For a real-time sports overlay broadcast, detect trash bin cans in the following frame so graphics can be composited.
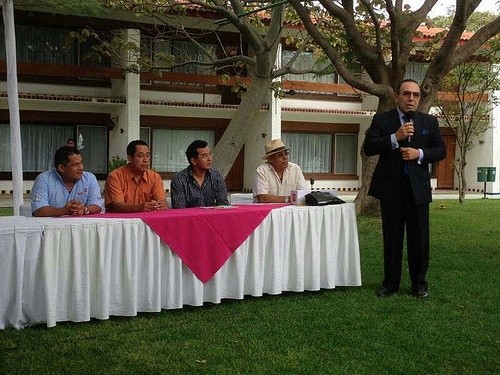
[477,166,496,183]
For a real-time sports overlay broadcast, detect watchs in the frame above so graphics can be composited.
[285,196,290,203]
[83,205,90,215]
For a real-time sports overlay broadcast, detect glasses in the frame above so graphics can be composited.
[197,151,214,158]
[397,91,421,98]
[274,151,289,158]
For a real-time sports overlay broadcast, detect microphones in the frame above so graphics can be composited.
[406,110,416,142]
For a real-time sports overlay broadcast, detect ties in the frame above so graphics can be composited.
[402,116,412,174]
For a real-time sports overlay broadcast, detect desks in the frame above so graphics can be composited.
[0,202,363,330]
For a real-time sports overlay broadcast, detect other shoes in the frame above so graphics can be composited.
[414,289,428,298]
[377,288,395,296]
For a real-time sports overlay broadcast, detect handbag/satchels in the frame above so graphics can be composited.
[305,191,334,205]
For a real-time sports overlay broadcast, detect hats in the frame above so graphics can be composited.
[262,138,290,159]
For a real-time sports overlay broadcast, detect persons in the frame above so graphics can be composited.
[363,79,447,298]
[67,138,76,147]
[30,145,105,217]
[171,140,229,209]
[252,139,305,204]
[103,140,169,214]
